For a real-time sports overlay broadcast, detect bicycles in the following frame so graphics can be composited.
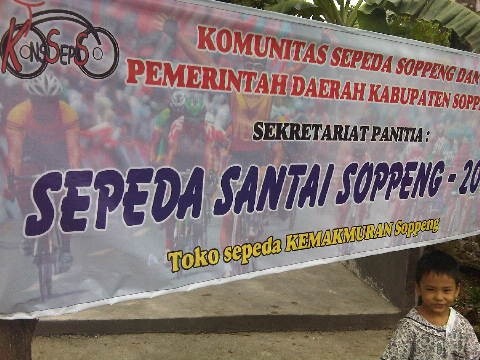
[223,166,480,280]
[166,168,215,266]
[8,165,69,301]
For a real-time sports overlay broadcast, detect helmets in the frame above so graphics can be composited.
[25,68,64,96]
[186,92,205,120]
[170,92,187,109]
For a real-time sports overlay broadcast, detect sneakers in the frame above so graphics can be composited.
[60,250,72,263]
[225,260,234,277]
[164,248,175,265]
[20,237,36,256]
[247,225,264,239]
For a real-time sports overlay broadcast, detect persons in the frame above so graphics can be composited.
[0,35,480,279]
[380,251,480,360]
